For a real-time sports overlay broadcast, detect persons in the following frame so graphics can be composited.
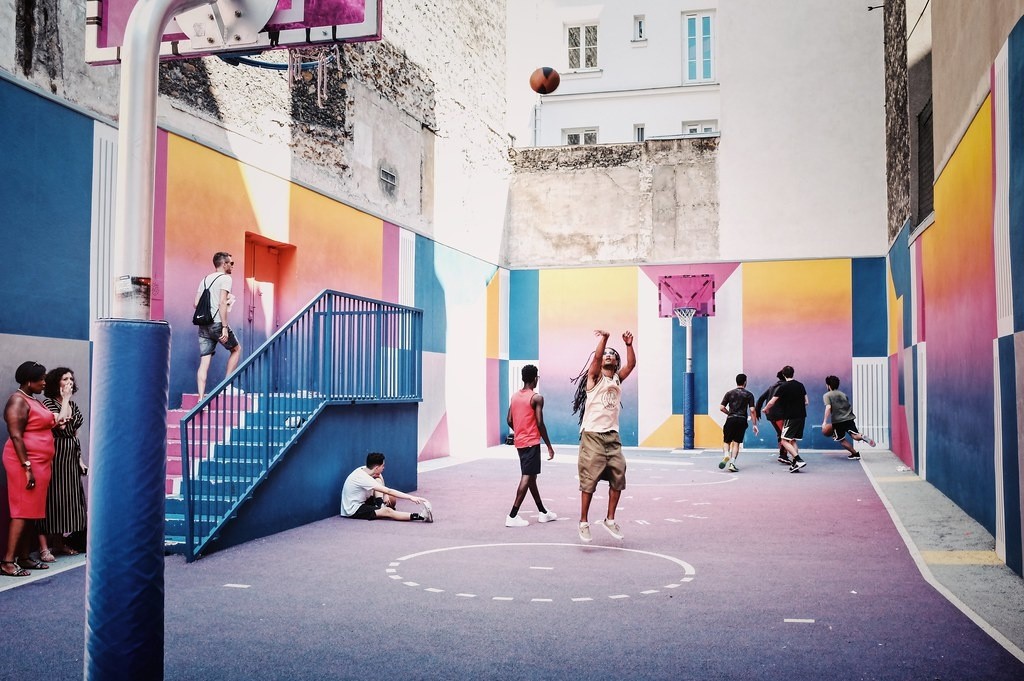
[720,374,759,471]
[504,365,557,527]
[341,453,434,523]
[0,361,56,577]
[570,330,636,543]
[822,375,876,459]
[42,367,89,561]
[194,252,245,410]
[755,370,792,464]
[762,366,809,472]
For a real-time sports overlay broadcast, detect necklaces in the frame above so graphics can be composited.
[17,388,36,400]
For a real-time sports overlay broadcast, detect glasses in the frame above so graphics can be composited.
[32,360,41,367]
[533,375,540,380]
[223,262,234,266]
[603,351,616,356]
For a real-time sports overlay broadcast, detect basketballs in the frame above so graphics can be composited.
[529,66,561,94]
[822,423,834,437]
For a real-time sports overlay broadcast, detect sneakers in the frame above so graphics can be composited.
[505,515,529,527]
[578,523,592,542]
[539,510,557,523]
[603,519,624,540]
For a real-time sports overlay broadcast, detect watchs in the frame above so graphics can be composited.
[22,461,31,467]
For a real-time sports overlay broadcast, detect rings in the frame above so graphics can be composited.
[32,483,34,486]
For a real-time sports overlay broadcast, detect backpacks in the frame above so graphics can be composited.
[192,274,226,325]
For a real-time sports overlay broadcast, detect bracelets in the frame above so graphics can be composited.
[626,343,632,346]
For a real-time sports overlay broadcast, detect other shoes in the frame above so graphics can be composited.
[718,456,730,469]
[60,545,78,555]
[782,465,799,473]
[39,549,56,562]
[848,452,860,460]
[197,401,208,411]
[220,385,244,396]
[420,502,434,522]
[728,463,739,472]
[861,435,876,447]
[790,460,806,473]
[778,455,792,465]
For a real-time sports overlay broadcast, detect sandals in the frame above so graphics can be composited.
[0,560,31,576]
[17,557,49,569]
